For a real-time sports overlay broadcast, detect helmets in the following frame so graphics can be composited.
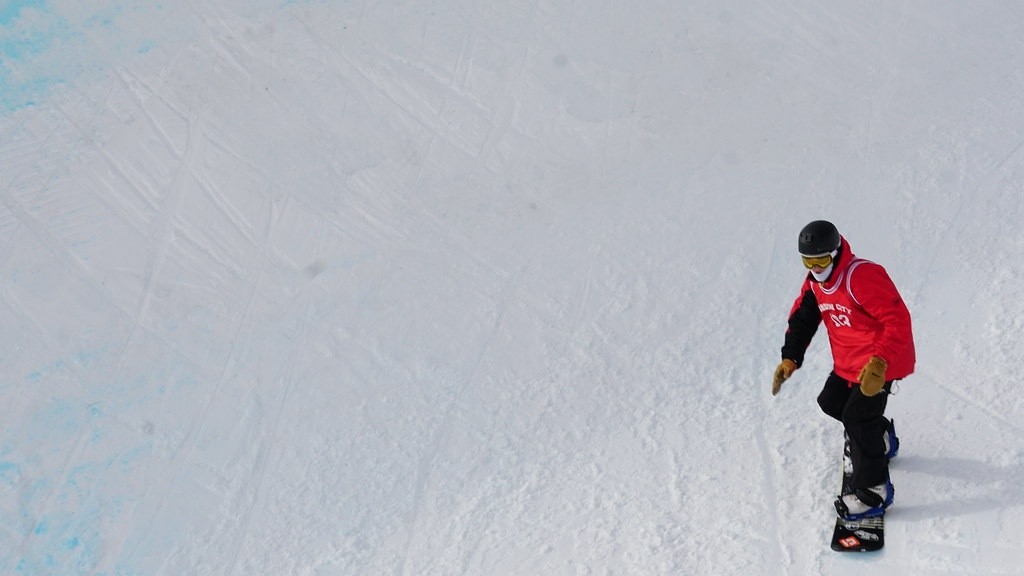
[797,220,840,256]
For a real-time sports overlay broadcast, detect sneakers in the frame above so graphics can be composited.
[842,437,892,465]
[840,478,894,516]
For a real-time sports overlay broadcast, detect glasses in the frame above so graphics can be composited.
[801,251,831,269]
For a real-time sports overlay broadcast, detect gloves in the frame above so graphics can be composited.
[857,356,887,396]
[771,358,797,395]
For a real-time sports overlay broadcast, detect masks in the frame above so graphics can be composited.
[810,264,833,283]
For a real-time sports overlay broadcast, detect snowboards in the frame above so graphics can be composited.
[828,420,888,553]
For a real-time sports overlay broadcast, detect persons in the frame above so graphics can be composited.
[772,220,917,518]
